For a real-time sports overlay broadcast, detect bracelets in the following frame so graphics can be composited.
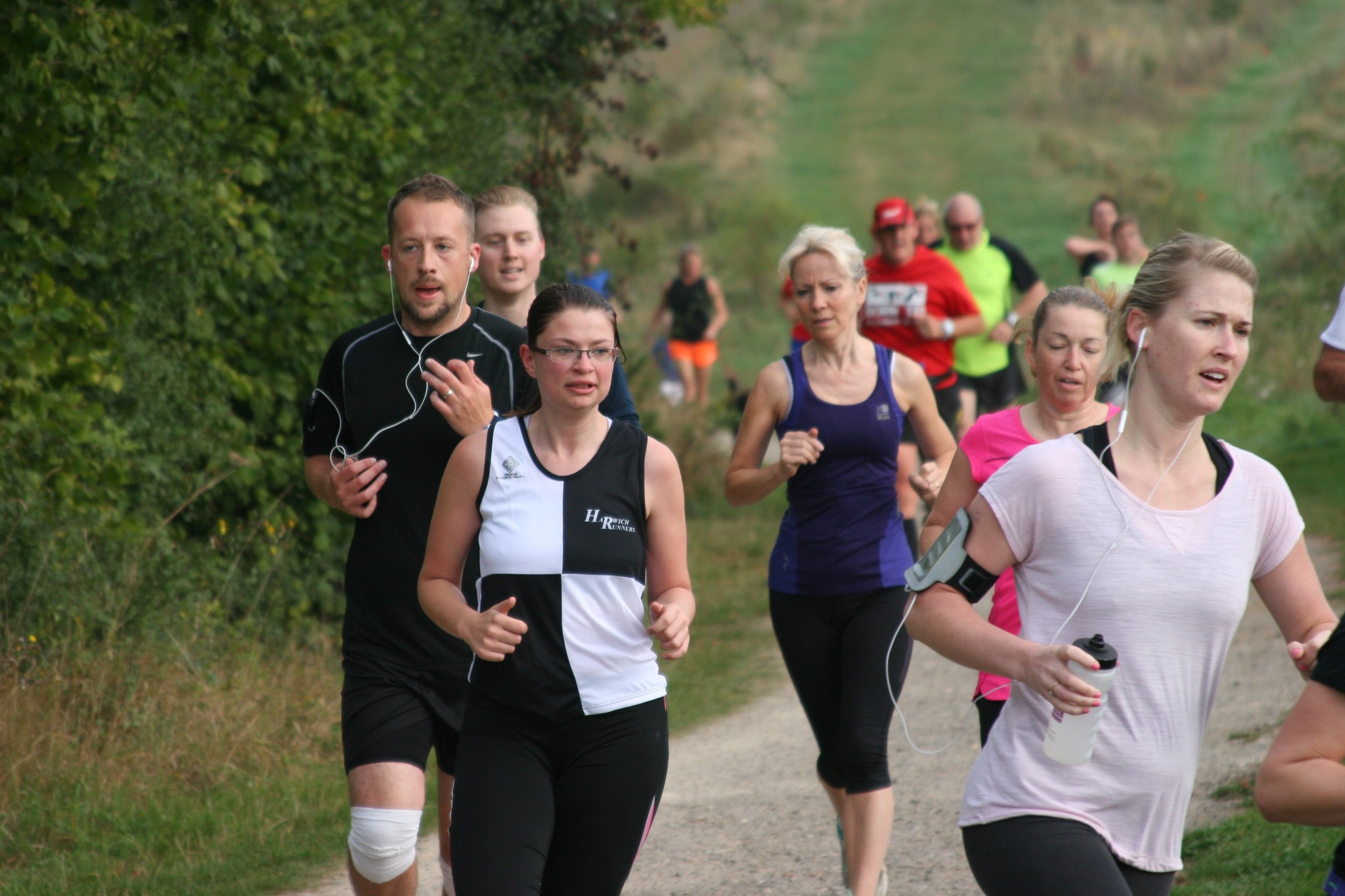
[940,317,955,341]
[484,409,499,430]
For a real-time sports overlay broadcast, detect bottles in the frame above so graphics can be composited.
[1041,633,1118,766]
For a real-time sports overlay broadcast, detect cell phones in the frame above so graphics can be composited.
[909,512,964,582]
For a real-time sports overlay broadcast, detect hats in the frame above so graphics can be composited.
[872,197,916,232]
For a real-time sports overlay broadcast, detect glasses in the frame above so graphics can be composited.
[945,221,983,232]
[530,346,621,364]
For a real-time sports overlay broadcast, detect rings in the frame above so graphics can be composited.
[442,389,453,399]
[1049,680,1061,697]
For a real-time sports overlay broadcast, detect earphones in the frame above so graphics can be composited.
[468,256,474,273]
[1137,324,1148,349]
[388,259,392,273]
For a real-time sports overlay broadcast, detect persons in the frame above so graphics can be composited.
[562,240,623,323]
[853,193,985,567]
[916,277,1131,752]
[469,184,642,429]
[1313,282,1345,403]
[724,222,959,895]
[415,279,696,896]
[1252,609,1345,896]
[644,245,731,415]
[776,186,1158,448]
[301,176,544,896]
[899,231,1341,896]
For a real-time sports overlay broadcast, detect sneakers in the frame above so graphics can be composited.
[835,814,889,896]
